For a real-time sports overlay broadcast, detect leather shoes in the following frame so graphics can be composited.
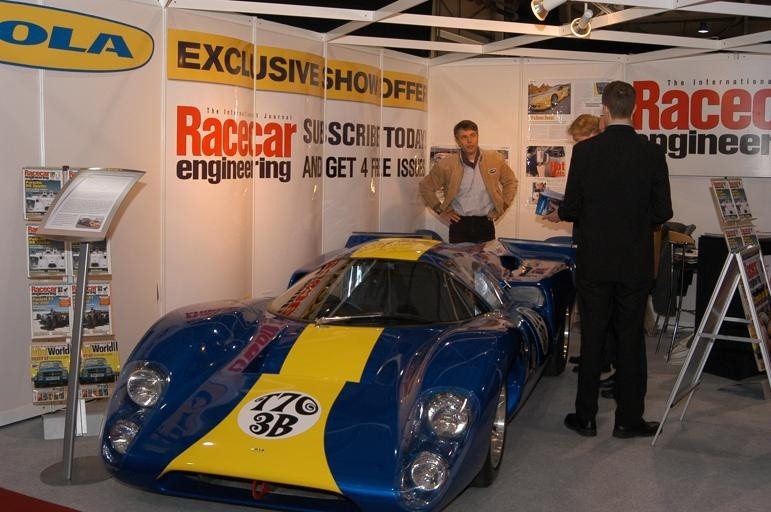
[613,422,662,438]
[564,414,597,436]
[569,356,615,399]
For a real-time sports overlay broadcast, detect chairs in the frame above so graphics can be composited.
[655,222,698,362]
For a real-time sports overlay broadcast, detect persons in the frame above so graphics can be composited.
[543,80,664,439]
[559,114,611,374]
[530,147,545,176]
[419,119,518,243]
[589,129,663,399]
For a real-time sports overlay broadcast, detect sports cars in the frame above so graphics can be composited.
[100,230,577,509]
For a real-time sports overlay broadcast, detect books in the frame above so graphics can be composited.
[535,189,566,215]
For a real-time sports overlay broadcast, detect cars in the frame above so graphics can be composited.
[34,360,68,387]
[79,358,115,385]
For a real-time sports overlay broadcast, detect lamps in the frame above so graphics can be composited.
[697,23,710,34]
[531,0,594,38]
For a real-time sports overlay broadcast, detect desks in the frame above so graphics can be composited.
[695,233,771,382]
